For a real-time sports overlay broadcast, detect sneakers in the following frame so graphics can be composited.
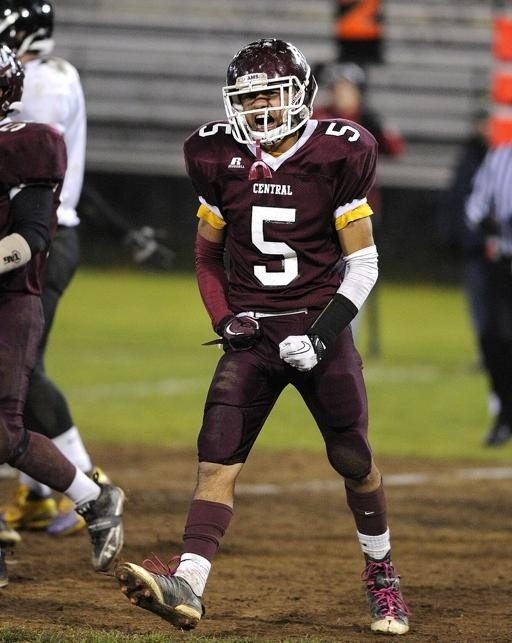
[360,550,412,635]
[1,464,204,631]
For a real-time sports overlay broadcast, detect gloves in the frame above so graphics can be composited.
[222,316,260,352]
[279,335,327,372]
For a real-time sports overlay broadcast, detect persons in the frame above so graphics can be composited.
[436,109,501,413]
[312,65,405,358]
[114,36,410,635]
[2,1,112,538]
[0,44,126,588]
[464,135,512,448]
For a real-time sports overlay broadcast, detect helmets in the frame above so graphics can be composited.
[221,37,319,145]
[0,41,24,116]
[0,0,54,55]
